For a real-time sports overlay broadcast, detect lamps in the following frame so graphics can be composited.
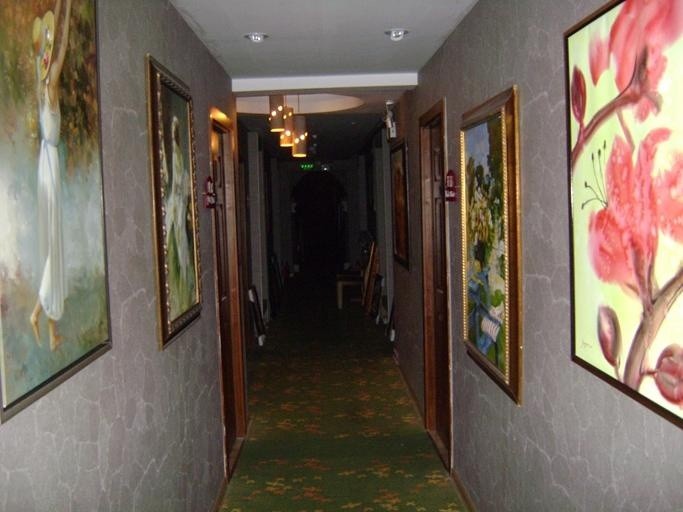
[265,87,309,160]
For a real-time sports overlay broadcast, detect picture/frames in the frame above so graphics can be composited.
[391,136,411,271]
[558,1,683,435]
[455,81,525,406]
[143,52,205,355]
[0,0,114,432]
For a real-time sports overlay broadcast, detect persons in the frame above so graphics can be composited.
[27,0,74,352]
[169,116,192,310]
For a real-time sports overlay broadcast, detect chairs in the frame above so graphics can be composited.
[334,241,374,309]
